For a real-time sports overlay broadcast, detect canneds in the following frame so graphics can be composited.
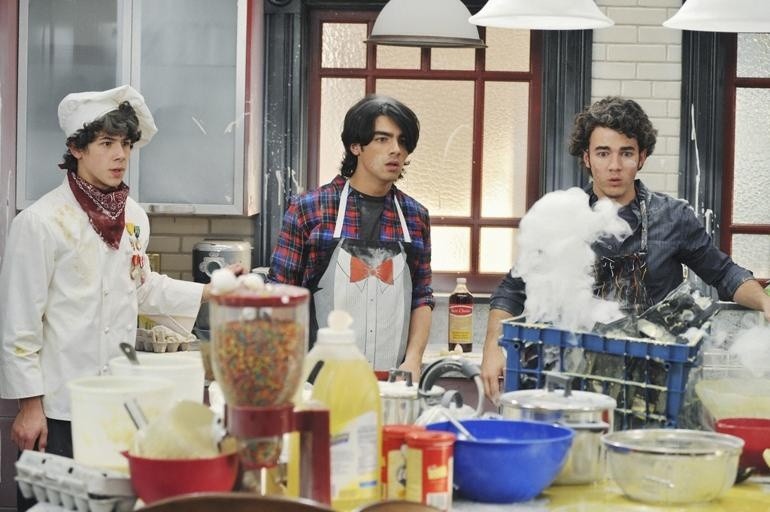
[405,429,454,510]
[382,424,423,501]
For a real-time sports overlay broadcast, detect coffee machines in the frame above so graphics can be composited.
[207,280,331,508]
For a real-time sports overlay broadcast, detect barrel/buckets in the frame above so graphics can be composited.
[293,328,383,510]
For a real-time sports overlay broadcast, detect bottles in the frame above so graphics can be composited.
[383,427,453,509]
[448,276,474,351]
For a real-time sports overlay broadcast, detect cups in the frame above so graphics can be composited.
[134,327,200,353]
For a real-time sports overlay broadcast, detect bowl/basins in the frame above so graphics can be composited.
[718,416,770,470]
[604,427,746,507]
[116,442,241,504]
[424,420,574,502]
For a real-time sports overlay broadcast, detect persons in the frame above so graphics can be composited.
[266,99,435,426]
[481,96,770,431]
[1,82,263,510]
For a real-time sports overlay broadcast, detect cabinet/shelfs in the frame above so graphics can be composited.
[15,2,264,218]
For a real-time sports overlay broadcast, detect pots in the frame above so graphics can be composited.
[496,372,619,482]
[375,369,446,425]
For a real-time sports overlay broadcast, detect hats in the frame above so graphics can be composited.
[57,86,158,149]
